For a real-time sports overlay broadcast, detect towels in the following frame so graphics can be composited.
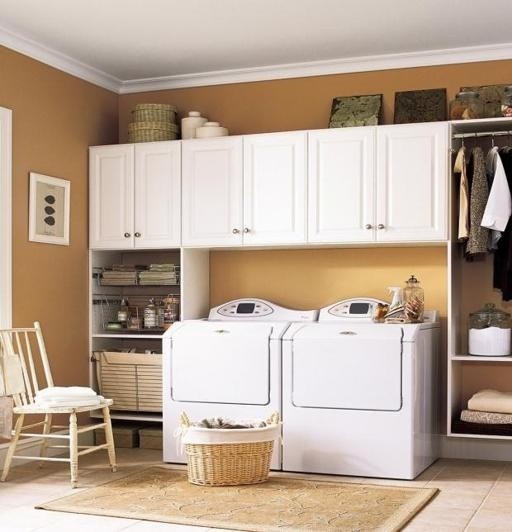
[34,385,103,410]
[0,330,26,396]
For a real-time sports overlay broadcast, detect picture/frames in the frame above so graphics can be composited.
[327,92,383,128]
[393,87,446,124]
[27,170,71,245]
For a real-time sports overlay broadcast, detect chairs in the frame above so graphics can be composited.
[0,321,118,488]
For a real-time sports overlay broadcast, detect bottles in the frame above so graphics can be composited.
[402,272,425,324]
[117,297,164,329]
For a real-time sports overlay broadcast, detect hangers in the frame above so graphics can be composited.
[461,129,510,148]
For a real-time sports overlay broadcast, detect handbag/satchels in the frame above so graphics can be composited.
[0,353,25,397]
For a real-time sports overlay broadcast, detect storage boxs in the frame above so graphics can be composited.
[138,428,163,450]
[94,426,140,449]
[94,351,162,413]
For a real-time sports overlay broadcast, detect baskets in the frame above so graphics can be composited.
[131,103,178,123]
[180,409,279,487]
[126,122,179,143]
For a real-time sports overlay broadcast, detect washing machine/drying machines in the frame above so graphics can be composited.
[162,297,318,470]
[281,298,442,480]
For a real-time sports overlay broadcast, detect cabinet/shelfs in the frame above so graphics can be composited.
[182,129,308,249]
[446,117,512,440]
[87,249,209,421]
[307,120,449,248]
[88,139,182,249]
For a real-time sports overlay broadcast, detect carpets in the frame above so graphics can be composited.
[33,465,439,532]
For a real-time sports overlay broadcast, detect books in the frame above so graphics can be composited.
[100,262,180,285]
